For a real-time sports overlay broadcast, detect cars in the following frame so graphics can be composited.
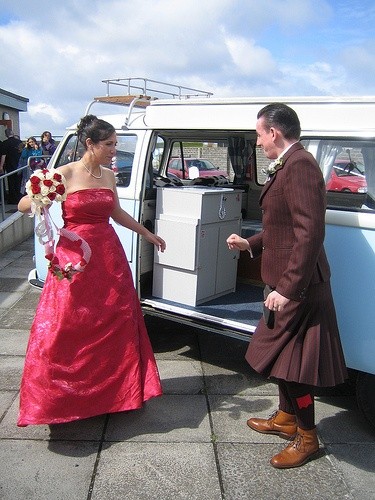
[326,158,368,197]
[164,155,232,185]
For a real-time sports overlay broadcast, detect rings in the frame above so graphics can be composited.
[273,306,278,309]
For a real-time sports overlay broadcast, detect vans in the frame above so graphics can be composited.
[27,73,374,415]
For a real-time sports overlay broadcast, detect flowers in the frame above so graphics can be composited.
[25,166,92,283]
[261,160,283,185]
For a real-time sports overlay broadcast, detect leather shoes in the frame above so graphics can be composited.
[270,426,319,468]
[247,410,297,440]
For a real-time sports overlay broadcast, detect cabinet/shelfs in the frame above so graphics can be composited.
[151,187,245,308]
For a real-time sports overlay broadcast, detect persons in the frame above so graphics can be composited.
[226,103,350,469]
[0,128,82,205]
[16,114,166,428]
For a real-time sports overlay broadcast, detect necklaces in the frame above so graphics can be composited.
[81,158,103,179]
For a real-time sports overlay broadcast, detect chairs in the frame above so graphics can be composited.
[152,177,184,188]
[189,175,229,186]
